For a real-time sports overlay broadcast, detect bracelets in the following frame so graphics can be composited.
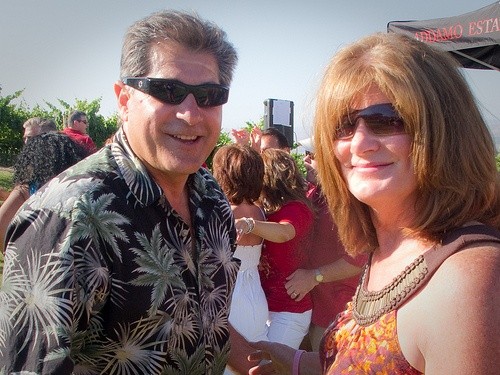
[258,360,269,366]
[248,217,256,232]
[242,216,252,234]
[292,350,307,375]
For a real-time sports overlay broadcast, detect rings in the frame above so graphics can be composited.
[293,292,298,296]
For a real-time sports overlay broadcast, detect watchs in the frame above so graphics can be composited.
[315,268,324,283]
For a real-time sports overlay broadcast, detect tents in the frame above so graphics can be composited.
[386,0,500,71]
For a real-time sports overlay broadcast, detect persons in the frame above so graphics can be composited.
[200,121,370,375]
[246,33,500,375]
[164,85,176,101]
[0,8,271,375]
[0,109,115,254]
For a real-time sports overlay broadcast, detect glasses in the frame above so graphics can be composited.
[78,120,87,124]
[124,77,229,107]
[334,103,410,140]
[305,150,318,159]
